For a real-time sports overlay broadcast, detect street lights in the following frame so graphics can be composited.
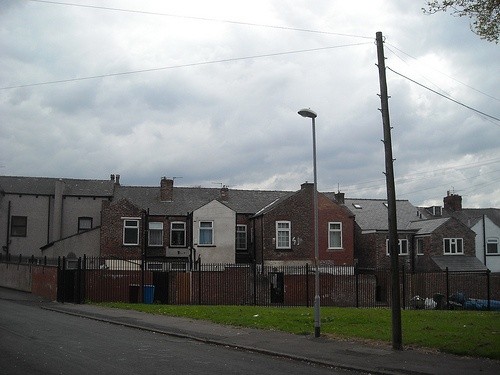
[297,109,321,340]
